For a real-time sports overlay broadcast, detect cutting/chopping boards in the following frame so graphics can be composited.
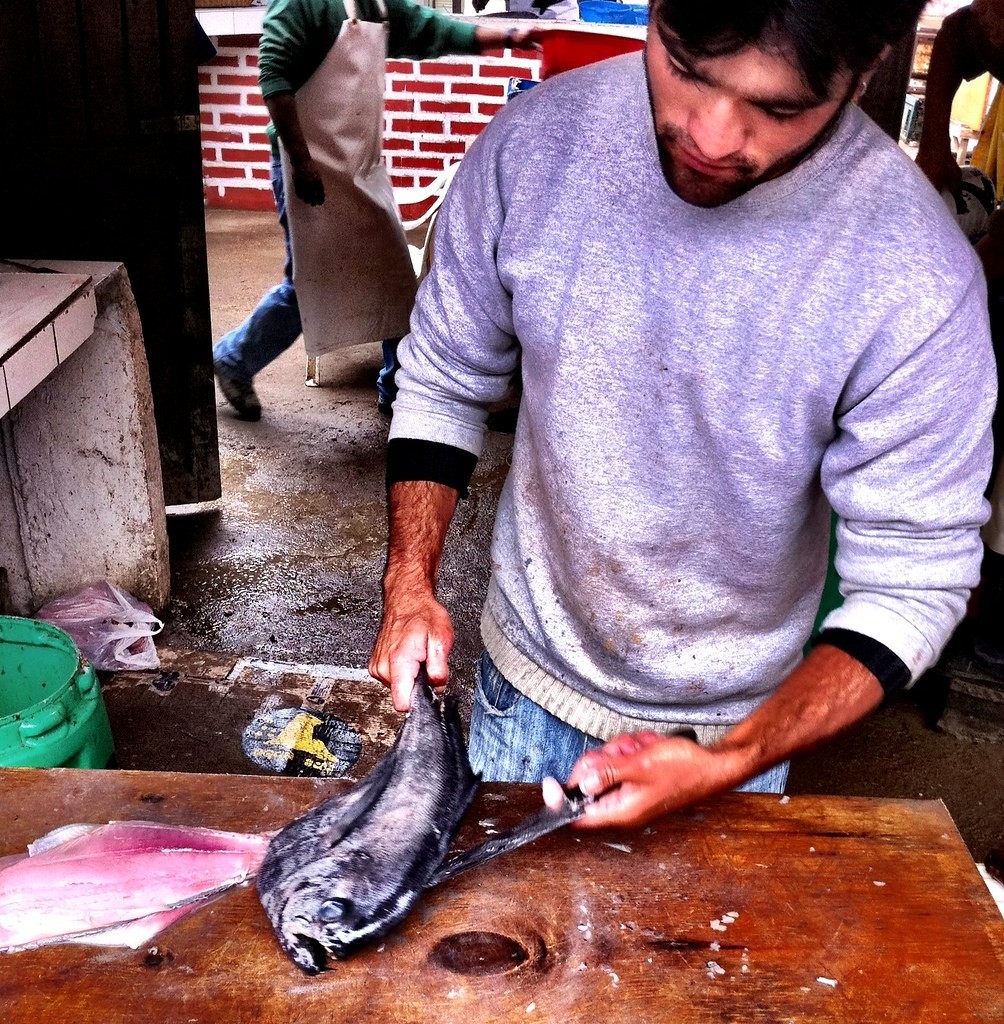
[0,767,1004,1024]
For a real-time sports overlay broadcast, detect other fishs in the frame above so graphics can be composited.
[256,662,483,977]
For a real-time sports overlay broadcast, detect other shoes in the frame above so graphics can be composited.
[213,354,261,416]
[378,401,393,412]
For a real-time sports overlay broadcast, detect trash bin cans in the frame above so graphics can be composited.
[0,613,115,770]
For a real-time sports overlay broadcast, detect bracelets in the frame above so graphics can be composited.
[505,28,517,49]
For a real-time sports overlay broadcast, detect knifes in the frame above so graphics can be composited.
[429,727,696,915]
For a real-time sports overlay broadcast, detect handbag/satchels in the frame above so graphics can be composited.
[38,581,166,670]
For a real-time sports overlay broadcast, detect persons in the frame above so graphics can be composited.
[212,0,558,420]
[367,0,1004,827]
